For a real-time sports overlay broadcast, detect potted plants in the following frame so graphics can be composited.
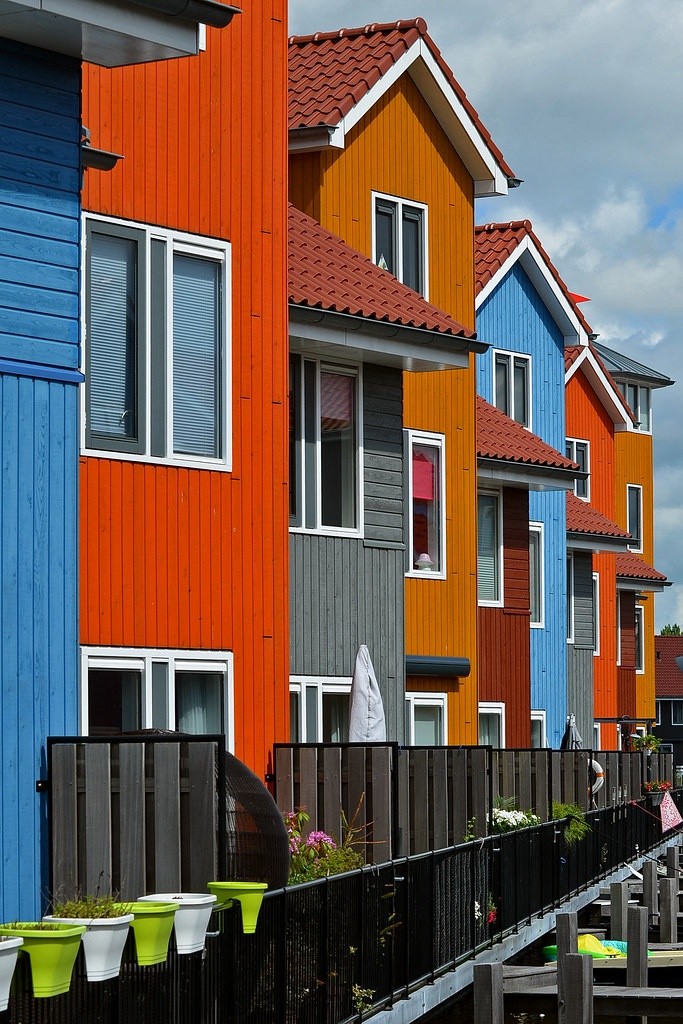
[0,917,86,998]
[42,870,134,982]
[649,791,666,807]
[633,734,664,755]
[0,934,24,1011]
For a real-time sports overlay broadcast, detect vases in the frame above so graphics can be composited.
[137,893,217,954]
[96,902,180,965]
[207,882,268,933]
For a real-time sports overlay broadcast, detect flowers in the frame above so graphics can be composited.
[640,780,671,791]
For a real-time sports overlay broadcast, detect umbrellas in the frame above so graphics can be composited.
[560,715,583,750]
[343,644,386,742]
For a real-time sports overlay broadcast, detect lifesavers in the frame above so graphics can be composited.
[588,758,606,796]
